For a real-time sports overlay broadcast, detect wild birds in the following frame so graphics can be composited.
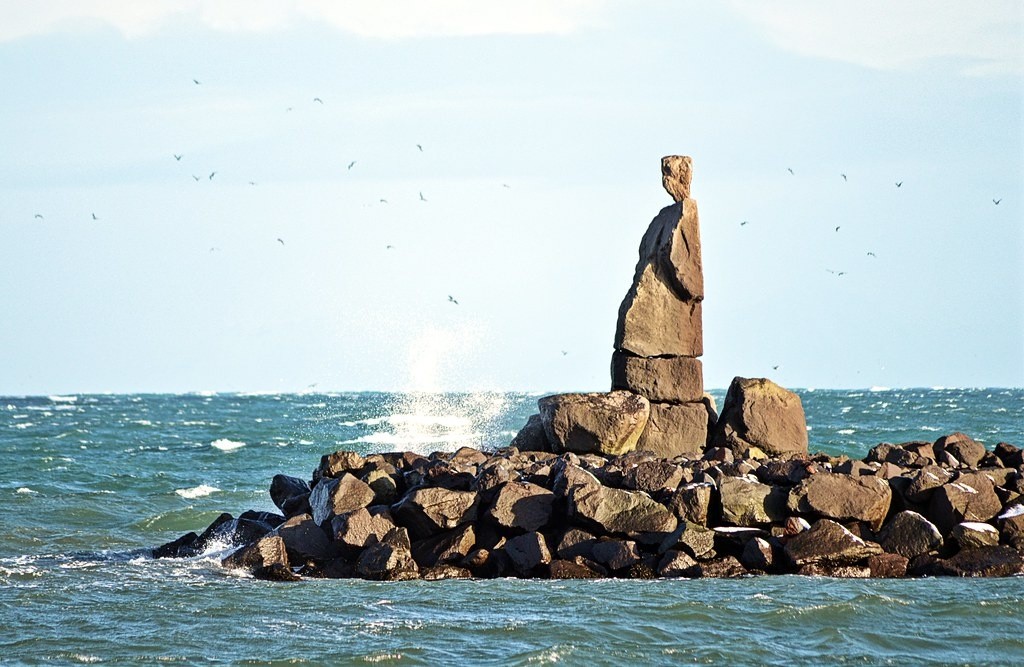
[740,164,1001,369]
[35,68,473,306]
